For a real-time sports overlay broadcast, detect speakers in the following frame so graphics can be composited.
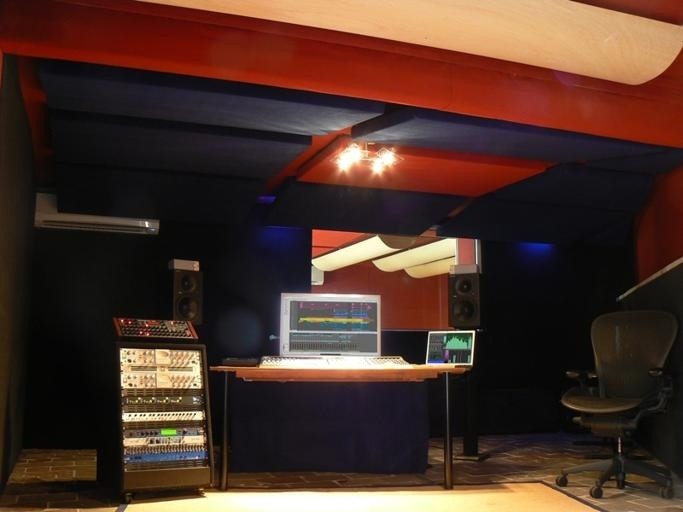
[447,272,481,328]
[172,269,204,326]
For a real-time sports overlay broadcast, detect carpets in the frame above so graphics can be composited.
[113,477,610,512]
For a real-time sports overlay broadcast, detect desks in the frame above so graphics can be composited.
[209,367,470,490]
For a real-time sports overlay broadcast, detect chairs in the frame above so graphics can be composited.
[555,308,676,501]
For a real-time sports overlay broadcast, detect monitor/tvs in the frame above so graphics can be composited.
[425,329,476,366]
[279,290,381,357]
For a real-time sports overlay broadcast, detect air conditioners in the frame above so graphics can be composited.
[33,192,160,237]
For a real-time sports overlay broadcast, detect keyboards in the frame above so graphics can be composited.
[258,353,414,370]
